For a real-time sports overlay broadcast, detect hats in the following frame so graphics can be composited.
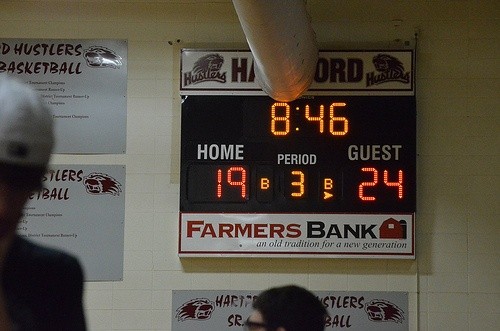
[0,75,53,166]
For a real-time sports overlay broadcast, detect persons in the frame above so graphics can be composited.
[246,285,327,331]
[0,74,88,331]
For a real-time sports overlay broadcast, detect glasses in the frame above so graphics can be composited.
[245,316,268,331]
